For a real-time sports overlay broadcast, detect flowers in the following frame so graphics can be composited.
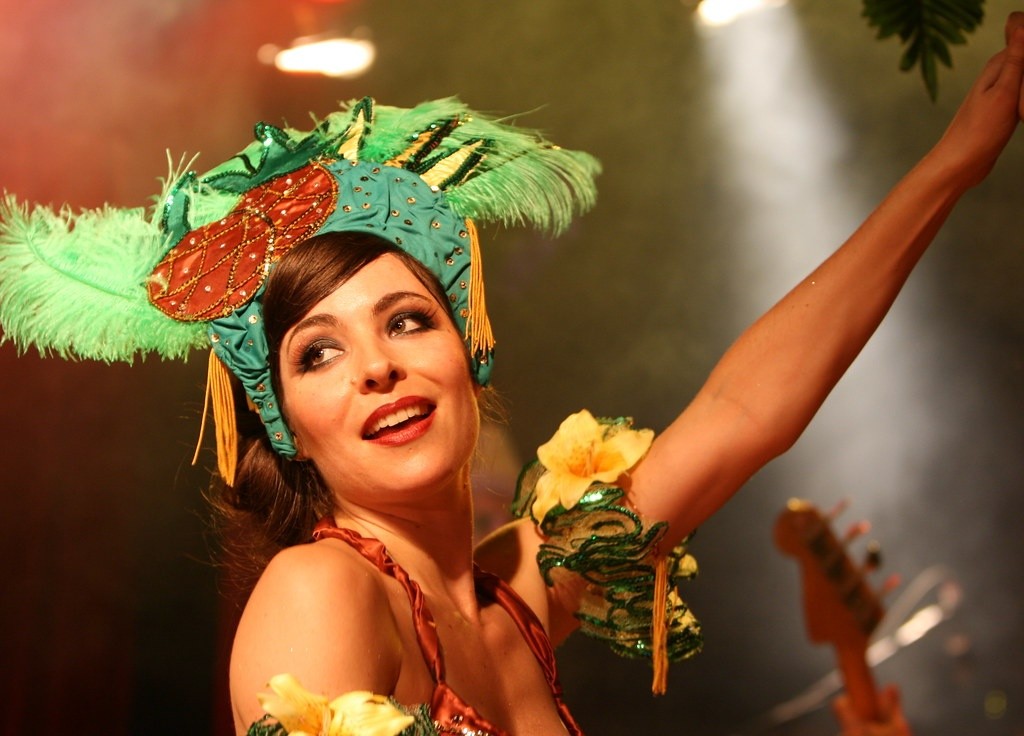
[253,674,417,736]
[526,407,655,527]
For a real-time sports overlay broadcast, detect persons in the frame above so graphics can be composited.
[0,0,1024,736]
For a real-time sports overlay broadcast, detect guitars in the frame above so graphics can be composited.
[774,492,917,736]
